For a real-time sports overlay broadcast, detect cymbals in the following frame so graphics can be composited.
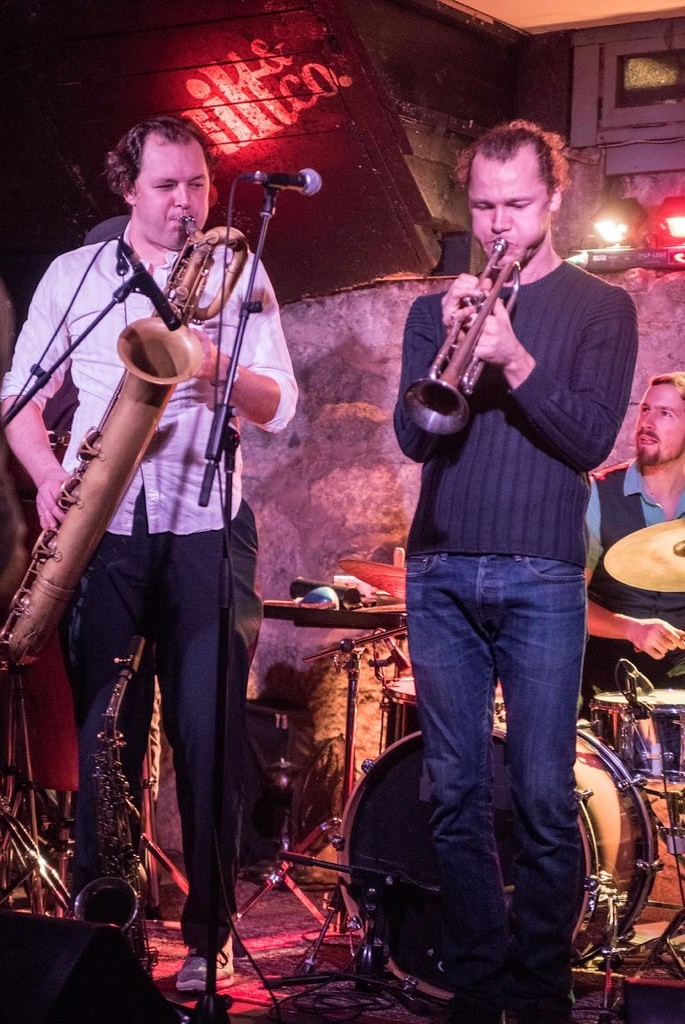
[338,559,407,601]
[602,516,684,592]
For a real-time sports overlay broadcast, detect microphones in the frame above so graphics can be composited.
[242,167,322,196]
[117,239,182,332]
[621,660,656,695]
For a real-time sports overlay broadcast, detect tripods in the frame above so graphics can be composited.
[0,669,64,914]
[260,850,443,1015]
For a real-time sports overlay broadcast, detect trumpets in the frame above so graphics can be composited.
[403,235,522,435]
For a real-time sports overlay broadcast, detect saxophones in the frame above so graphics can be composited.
[0,215,252,672]
[74,640,155,979]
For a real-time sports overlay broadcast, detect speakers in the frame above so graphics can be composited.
[0,907,185,1024]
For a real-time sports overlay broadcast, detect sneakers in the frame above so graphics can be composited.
[175,937,235,991]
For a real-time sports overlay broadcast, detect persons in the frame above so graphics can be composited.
[581,372,685,721]
[0,115,300,995]
[391,117,638,1024]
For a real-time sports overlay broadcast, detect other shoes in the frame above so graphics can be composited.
[449,1005,502,1024]
[515,1009,577,1024]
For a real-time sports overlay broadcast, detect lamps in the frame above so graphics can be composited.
[651,194,685,249]
[591,196,648,250]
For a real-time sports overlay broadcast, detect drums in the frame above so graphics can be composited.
[335,716,661,1001]
[589,684,685,782]
[384,678,506,749]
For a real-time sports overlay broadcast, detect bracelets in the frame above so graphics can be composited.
[210,355,239,391]
